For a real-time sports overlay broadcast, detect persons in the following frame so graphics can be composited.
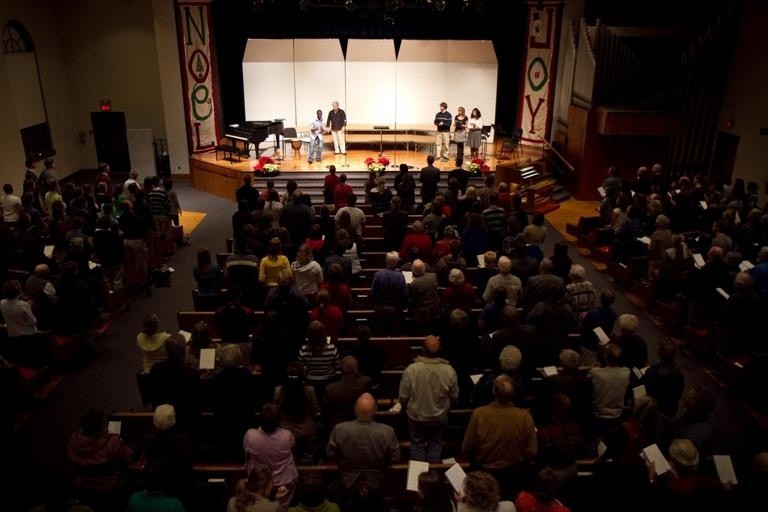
[326,101,348,155]
[601,162,767,327]
[1,156,183,343]
[307,110,325,163]
[433,102,452,162]
[465,108,483,160]
[193,158,617,344]
[453,107,469,166]
[66,313,767,509]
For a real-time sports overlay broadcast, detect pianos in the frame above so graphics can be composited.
[224,121,284,159]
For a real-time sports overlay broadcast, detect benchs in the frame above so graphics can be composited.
[215,144,241,162]
[0,204,767,512]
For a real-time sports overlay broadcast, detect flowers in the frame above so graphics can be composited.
[364,157,390,175]
[466,159,490,177]
[254,156,278,176]
